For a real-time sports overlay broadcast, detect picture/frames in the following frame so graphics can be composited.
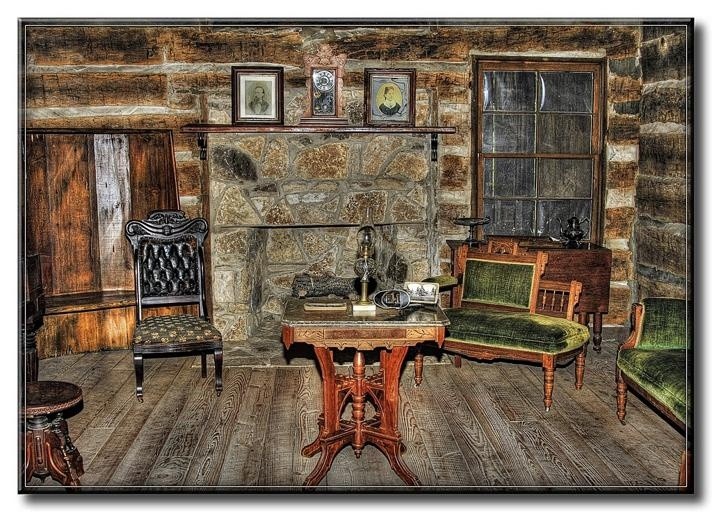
[230,65,286,126]
[363,67,417,129]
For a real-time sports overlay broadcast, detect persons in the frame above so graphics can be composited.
[248,86,269,115]
[379,86,402,116]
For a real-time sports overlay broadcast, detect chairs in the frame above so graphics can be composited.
[124,207,226,403]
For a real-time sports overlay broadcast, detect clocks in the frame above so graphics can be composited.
[310,65,340,116]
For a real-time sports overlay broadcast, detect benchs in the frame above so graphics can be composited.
[614,296,690,492]
[420,249,591,412]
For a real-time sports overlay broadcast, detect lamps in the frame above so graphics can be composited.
[352,226,380,310]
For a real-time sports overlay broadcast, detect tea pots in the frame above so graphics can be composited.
[555,215,592,251]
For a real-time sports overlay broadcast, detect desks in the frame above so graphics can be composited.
[22,380,87,490]
[281,295,449,491]
[446,235,612,353]
